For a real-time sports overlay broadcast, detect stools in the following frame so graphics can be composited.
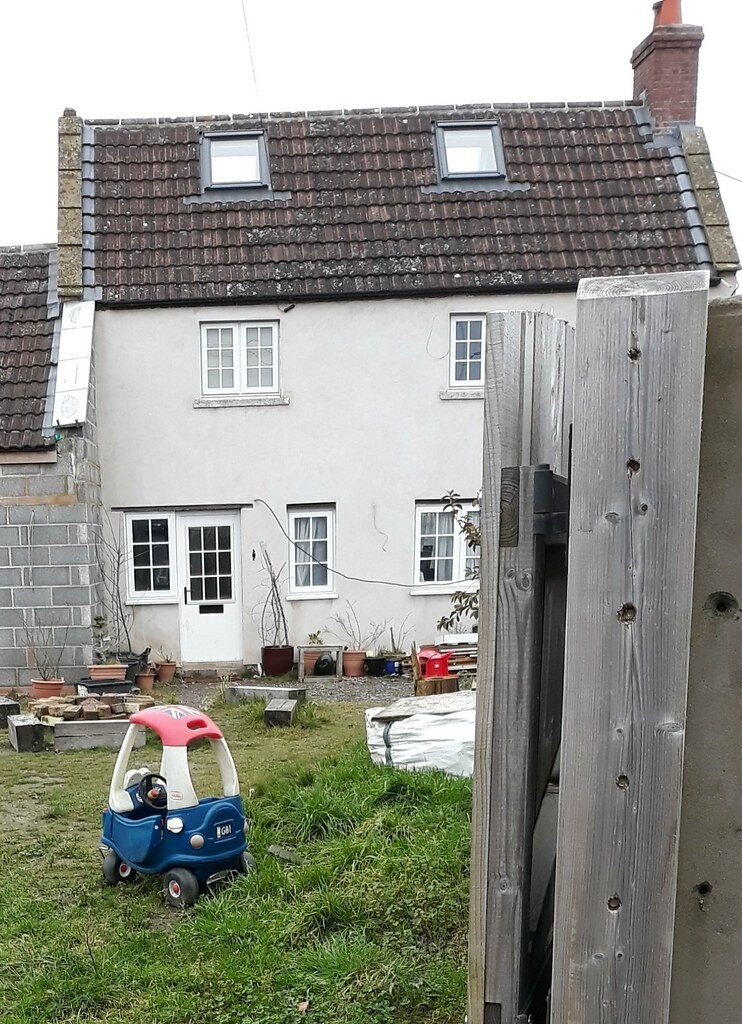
[297,644,343,683]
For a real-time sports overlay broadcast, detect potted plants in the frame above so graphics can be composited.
[324,598,392,676]
[384,612,417,675]
[152,645,177,682]
[18,614,70,698]
[88,657,129,680]
[252,541,294,677]
[362,644,387,676]
[91,497,150,681]
[134,662,160,690]
[304,630,328,673]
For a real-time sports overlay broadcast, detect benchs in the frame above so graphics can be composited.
[264,699,298,727]
[53,720,146,750]
[7,714,44,752]
[0,695,20,728]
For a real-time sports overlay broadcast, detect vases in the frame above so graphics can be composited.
[73,678,132,696]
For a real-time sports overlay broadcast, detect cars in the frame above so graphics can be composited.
[92,701,259,907]
